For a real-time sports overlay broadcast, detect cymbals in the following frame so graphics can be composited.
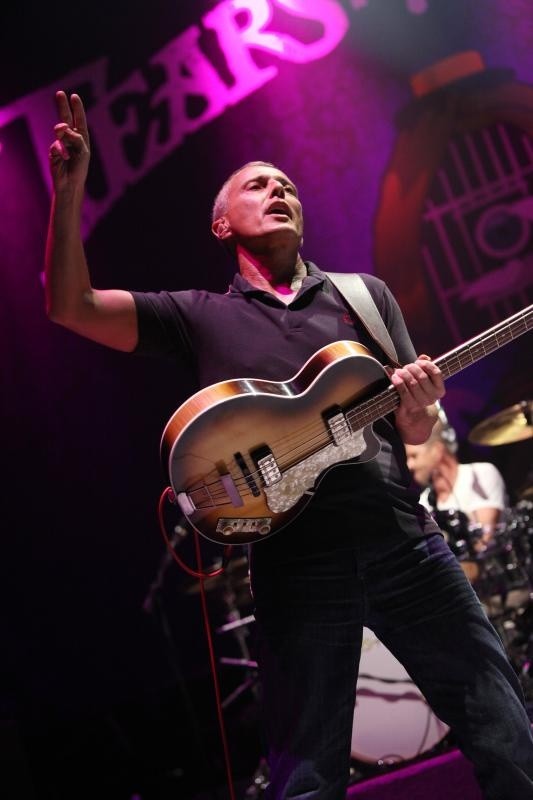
[466,399,533,448]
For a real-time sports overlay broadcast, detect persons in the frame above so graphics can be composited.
[44,90,533,800]
[403,399,507,583]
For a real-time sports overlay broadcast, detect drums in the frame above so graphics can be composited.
[351,628,451,767]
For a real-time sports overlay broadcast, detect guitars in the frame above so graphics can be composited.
[159,303,533,546]
